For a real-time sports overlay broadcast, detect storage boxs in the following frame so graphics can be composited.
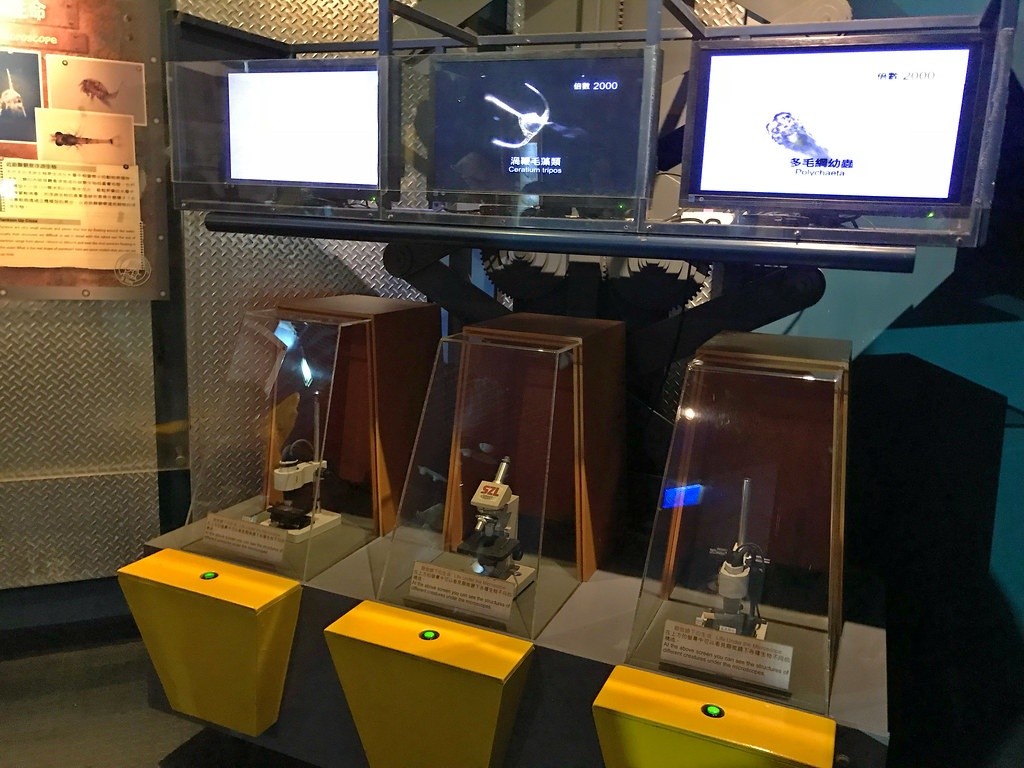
[376,327,585,636]
[181,306,379,582]
[616,360,848,716]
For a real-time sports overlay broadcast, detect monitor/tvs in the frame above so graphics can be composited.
[676,26,993,229]
[428,48,644,217]
[221,59,381,206]
